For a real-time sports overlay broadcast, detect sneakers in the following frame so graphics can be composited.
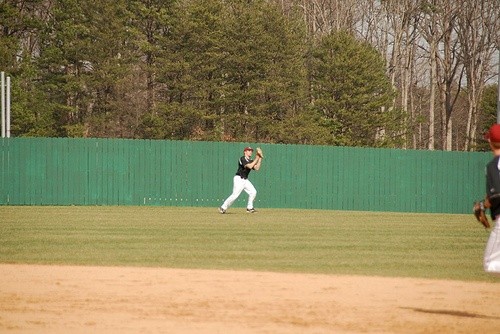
[247,208,256,213]
[218,207,225,214]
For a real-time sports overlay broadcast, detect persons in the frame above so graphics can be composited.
[473,124,500,274]
[219,147,263,214]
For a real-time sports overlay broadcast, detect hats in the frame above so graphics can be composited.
[244,147,253,151]
[484,124,500,142]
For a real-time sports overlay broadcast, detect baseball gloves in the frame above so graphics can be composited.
[256,147,263,158]
[472,199,491,229]
